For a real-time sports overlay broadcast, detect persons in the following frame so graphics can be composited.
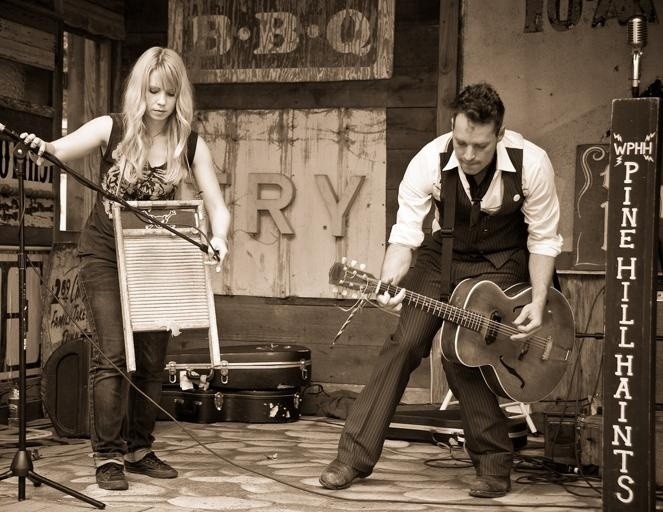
[314,80,563,500]
[14,46,230,493]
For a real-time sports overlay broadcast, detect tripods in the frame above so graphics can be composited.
[0,121,209,509]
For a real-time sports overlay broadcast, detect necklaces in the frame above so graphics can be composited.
[152,131,161,140]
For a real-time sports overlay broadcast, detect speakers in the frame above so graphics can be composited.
[40,337,93,439]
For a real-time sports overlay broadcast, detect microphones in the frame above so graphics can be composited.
[626,15,648,88]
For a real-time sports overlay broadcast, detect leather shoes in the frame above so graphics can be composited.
[319,458,374,490]
[124,451,178,479]
[468,473,510,498]
[95,462,128,490]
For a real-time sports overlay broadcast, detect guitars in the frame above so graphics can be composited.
[327,257,574,405]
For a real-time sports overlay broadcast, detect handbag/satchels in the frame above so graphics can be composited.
[154,343,312,424]
